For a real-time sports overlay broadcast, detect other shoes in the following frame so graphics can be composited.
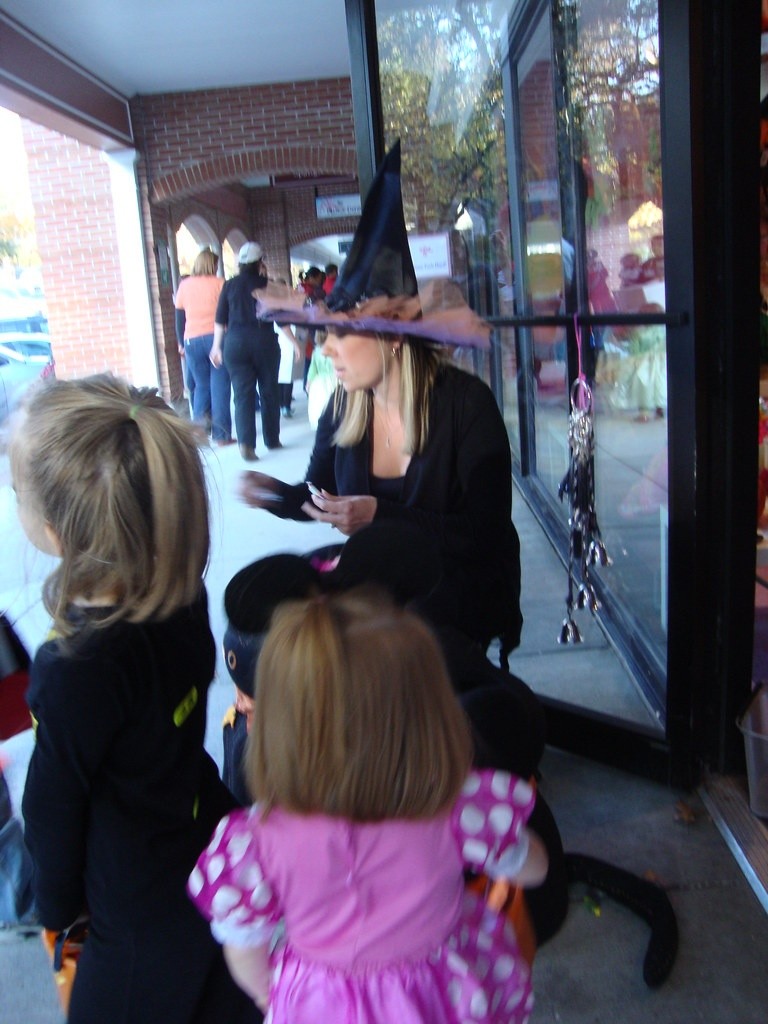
[267,442,283,451]
[283,407,294,419]
[240,443,259,461]
[218,439,237,448]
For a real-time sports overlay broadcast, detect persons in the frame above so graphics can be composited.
[274,264,339,431]
[0,614,42,944]
[585,236,665,422]
[175,250,236,447]
[186,590,551,1023]
[209,242,301,459]
[12,372,262,1024]
[226,291,524,634]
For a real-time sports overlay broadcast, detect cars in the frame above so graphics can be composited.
[0,347,54,425]
[1,332,50,360]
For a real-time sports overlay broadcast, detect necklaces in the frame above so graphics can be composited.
[386,436,389,446]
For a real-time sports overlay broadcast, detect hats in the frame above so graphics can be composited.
[239,242,264,263]
[257,137,493,348]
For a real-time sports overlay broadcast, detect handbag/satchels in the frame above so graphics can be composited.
[40,926,89,1014]
